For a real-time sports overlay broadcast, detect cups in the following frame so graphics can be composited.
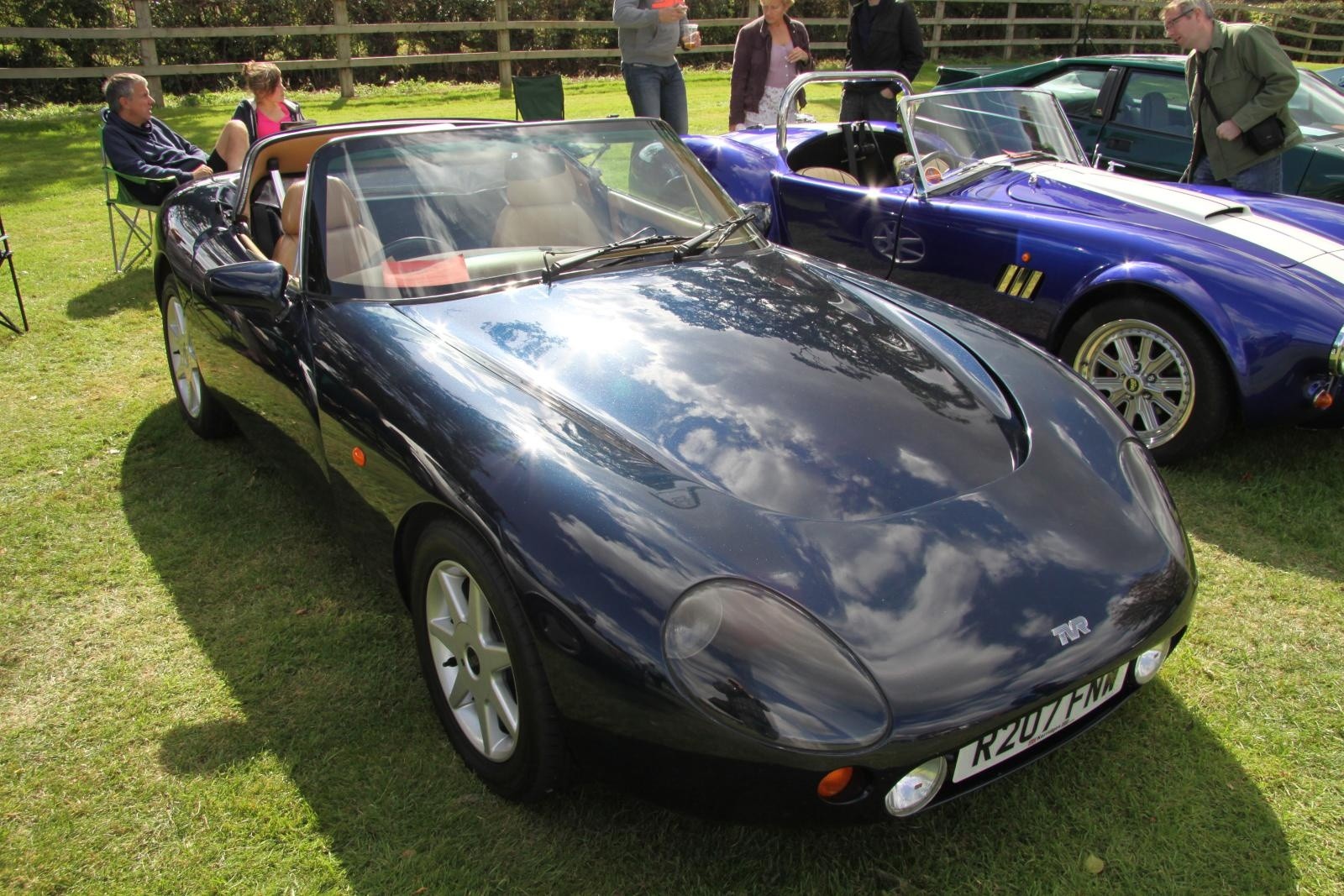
[682,24,699,49]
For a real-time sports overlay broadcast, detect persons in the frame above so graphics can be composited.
[729,0,814,132]
[612,0,700,134]
[230,62,306,143]
[102,73,249,206]
[838,0,925,123]
[1156,0,1305,194]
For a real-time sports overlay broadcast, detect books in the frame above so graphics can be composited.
[280,120,317,130]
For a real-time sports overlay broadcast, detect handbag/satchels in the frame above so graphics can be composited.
[1244,112,1287,156]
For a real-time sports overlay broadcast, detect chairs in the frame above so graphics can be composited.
[894,153,949,185]
[492,152,607,248]
[1141,92,1198,165]
[273,175,381,281]
[796,166,860,187]
[99,107,180,274]
[511,74,565,121]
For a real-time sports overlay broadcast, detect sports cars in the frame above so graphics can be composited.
[626,68,1344,467]
[911,52,1344,204]
[152,110,1201,831]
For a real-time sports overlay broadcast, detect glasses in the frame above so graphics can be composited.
[1164,6,1196,29]
